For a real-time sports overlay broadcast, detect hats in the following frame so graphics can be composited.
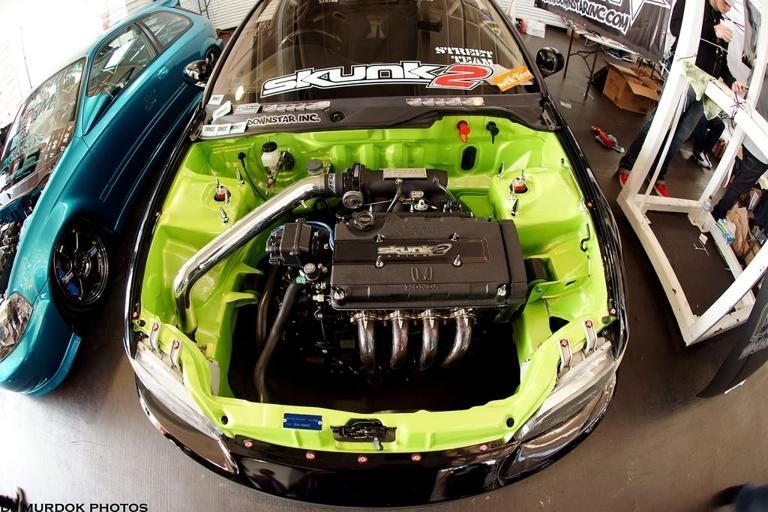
[722,0,741,14]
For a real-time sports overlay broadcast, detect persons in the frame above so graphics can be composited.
[713,480,767,511]
[617,0,768,277]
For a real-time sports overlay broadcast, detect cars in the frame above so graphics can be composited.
[0,0,231,401]
[122,1,631,509]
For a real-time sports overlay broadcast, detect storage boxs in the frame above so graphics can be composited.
[602,61,663,115]
[525,18,545,39]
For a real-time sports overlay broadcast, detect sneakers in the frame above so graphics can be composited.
[647,175,670,197]
[692,147,714,169]
[618,167,631,188]
